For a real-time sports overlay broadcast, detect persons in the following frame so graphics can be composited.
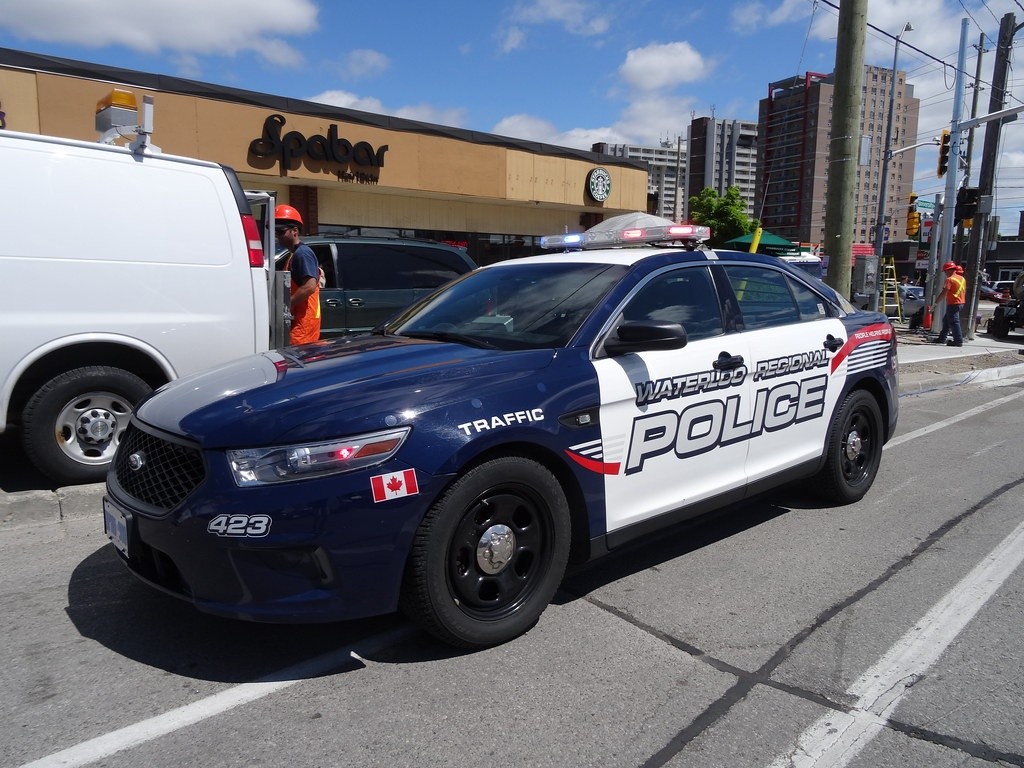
[930,262,966,347]
[265,204,323,345]
[892,276,908,324]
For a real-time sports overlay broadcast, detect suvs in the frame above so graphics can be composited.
[274,234,480,343]
[983,280,1014,291]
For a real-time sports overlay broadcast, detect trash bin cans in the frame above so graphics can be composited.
[991,307,1016,339]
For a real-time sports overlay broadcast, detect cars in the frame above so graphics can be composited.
[980,286,1005,302]
[101,227,901,649]
[854,281,927,314]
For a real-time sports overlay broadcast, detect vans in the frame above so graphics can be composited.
[1,89,271,485]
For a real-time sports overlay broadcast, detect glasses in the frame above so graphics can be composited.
[275,226,294,236]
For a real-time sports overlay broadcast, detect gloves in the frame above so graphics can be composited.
[930,303,937,310]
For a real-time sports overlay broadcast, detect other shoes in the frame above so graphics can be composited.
[947,341,962,347]
[932,338,946,344]
[899,321,907,324]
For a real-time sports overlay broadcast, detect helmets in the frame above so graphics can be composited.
[274,204,303,225]
[955,266,964,274]
[941,261,956,272]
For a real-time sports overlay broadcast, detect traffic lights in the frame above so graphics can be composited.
[907,193,919,235]
[937,131,951,179]
[954,186,977,227]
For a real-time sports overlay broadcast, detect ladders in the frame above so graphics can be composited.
[878,257,901,324]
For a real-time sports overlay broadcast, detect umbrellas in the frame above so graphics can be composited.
[725,230,798,249]
[582,212,683,247]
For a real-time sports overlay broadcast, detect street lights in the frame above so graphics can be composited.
[867,21,914,313]
